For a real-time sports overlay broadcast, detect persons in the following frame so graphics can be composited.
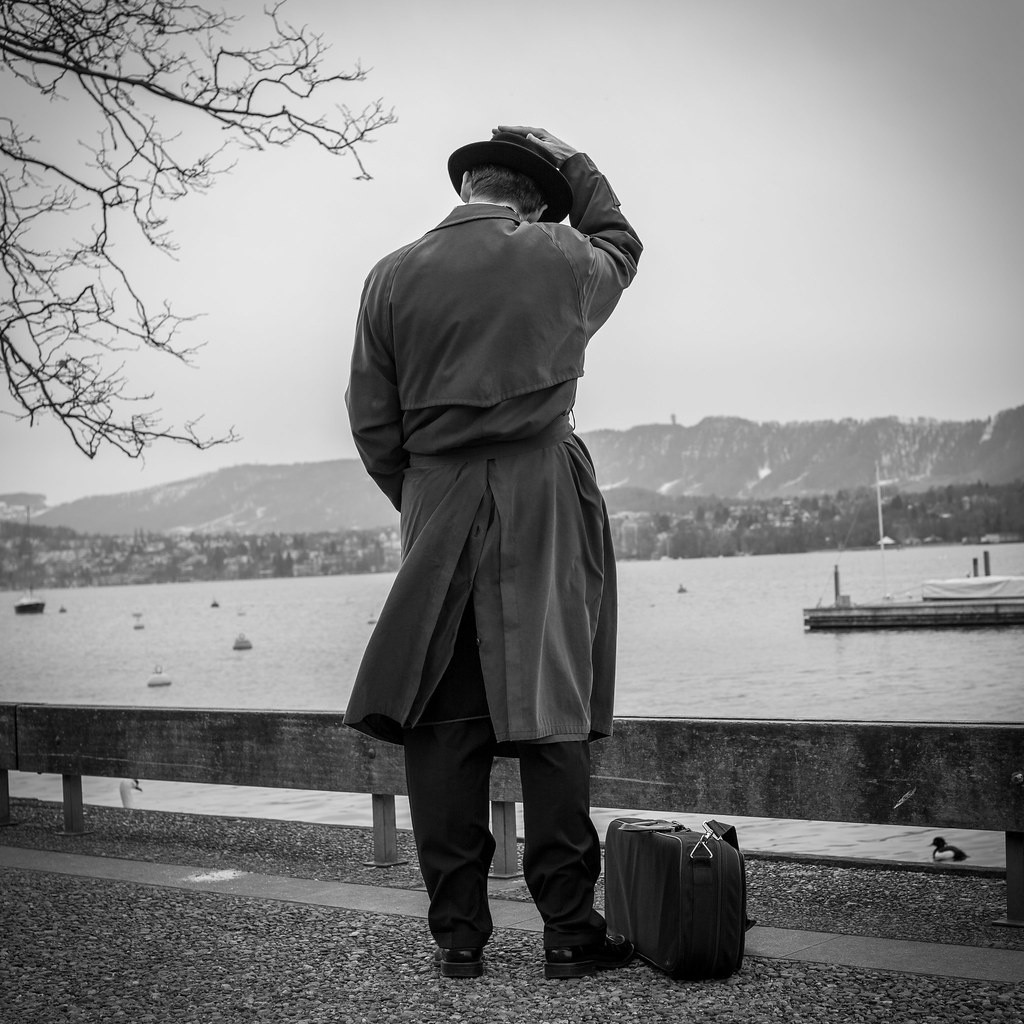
[342,123,644,977]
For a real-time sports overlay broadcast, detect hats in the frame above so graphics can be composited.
[448,132,574,223]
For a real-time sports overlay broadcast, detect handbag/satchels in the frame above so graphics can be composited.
[604,818,757,983]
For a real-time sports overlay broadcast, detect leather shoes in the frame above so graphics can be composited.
[544,933,636,980]
[433,947,484,977]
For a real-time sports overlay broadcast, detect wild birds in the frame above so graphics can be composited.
[926,828,971,862]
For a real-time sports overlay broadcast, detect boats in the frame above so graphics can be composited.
[800,551,1024,630]
